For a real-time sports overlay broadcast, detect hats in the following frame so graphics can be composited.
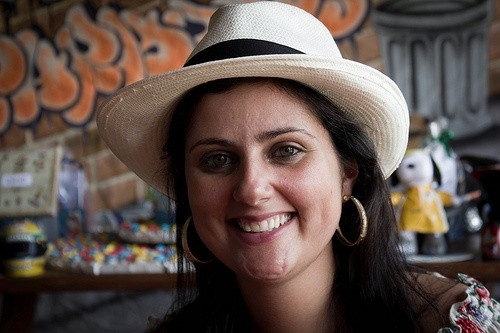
[96,2,410,202]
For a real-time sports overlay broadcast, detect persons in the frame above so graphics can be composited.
[140,1,500,333]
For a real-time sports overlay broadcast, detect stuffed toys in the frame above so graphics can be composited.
[386,148,481,257]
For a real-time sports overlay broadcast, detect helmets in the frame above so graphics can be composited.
[0,220,49,278]
